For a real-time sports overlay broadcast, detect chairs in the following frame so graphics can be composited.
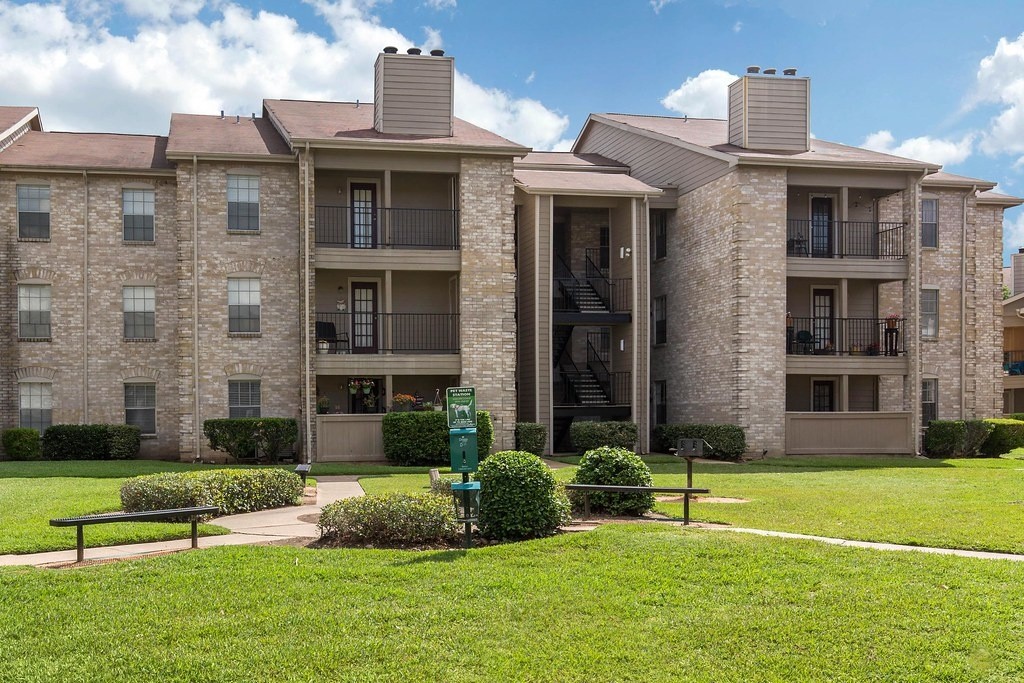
[796,330,813,354]
[315,321,350,354]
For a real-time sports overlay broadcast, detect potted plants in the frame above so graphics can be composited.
[423,401,443,411]
[318,396,330,414]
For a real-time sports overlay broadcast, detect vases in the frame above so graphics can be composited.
[887,319,896,328]
[869,349,879,356]
[786,318,793,326]
[350,388,358,394]
[826,351,834,355]
[318,341,329,353]
[391,405,413,412]
[363,388,370,394]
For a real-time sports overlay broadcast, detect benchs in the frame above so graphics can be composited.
[565,484,710,525]
[50,505,219,562]
[294,464,311,483]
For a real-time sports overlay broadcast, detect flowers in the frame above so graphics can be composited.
[868,343,880,349]
[348,380,360,389]
[786,312,792,318]
[849,344,862,351]
[886,314,900,319]
[394,393,416,406]
[361,380,374,389]
[825,343,833,350]
